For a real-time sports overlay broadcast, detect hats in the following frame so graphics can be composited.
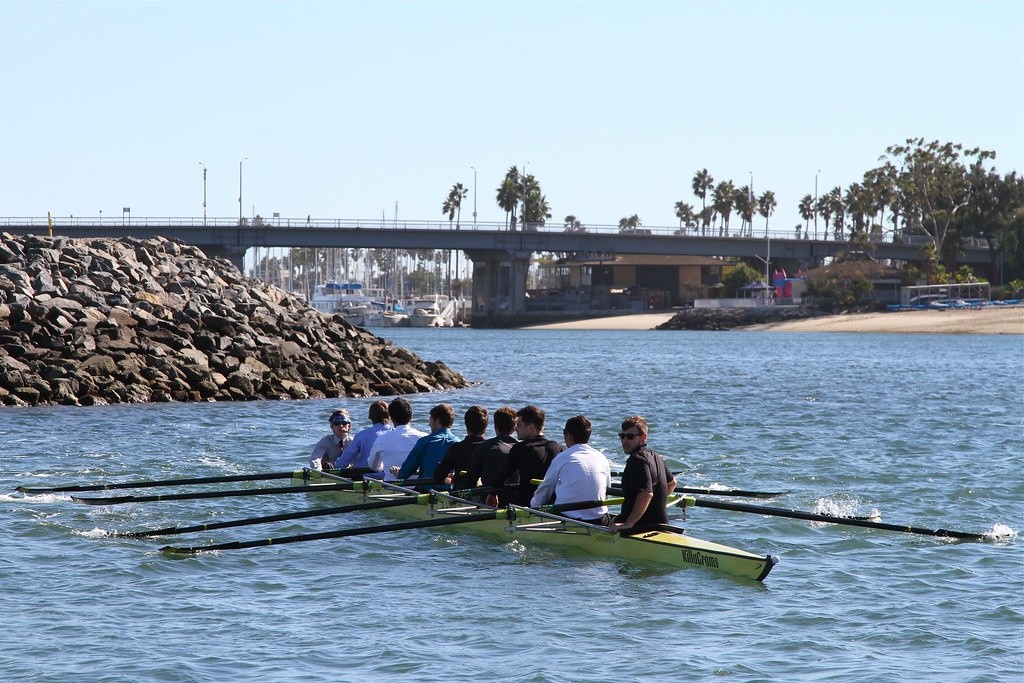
[370,401,389,415]
[331,413,352,422]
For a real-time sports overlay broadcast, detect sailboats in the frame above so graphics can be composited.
[312,202,460,327]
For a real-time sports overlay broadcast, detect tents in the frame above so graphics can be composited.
[736,281,773,298]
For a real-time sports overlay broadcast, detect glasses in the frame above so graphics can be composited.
[563,429,568,435]
[618,433,643,440]
[332,420,350,426]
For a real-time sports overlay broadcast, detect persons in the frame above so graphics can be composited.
[462,408,519,506]
[367,397,430,488]
[530,416,612,525]
[335,400,395,478]
[435,406,490,490]
[608,415,677,536]
[390,404,462,494]
[486,405,566,509]
[309,408,354,470]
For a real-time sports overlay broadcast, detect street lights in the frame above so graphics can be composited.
[750,172,753,238]
[199,162,206,226]
[471,167,476,230]
[240,157,248,226]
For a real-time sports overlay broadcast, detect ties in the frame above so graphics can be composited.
[337,439,345,459]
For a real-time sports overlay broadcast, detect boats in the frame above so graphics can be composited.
[291,467,780,582]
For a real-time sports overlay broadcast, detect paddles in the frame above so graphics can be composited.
[105,482,519,538]
[70,477,453,506]
[159,498,623,554]
[609,479,788,500]
[14,466,384,494]
[529,476,1012,540]
[389,467,685,475]
